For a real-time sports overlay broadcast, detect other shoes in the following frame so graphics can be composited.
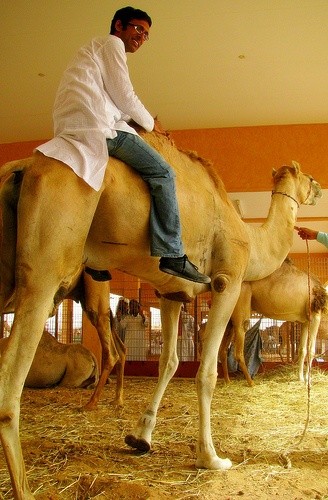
[159,256,211,283]
[84,267,112,282]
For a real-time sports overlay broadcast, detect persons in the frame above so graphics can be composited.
[177,309,200,361]
[114,297,148,361]
[52,6,211,284]
[268,330,283,362]
[298,226,328,248]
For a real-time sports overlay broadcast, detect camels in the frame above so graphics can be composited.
[0,116,328,500]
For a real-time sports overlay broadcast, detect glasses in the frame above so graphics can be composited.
[127,23,149,42]
